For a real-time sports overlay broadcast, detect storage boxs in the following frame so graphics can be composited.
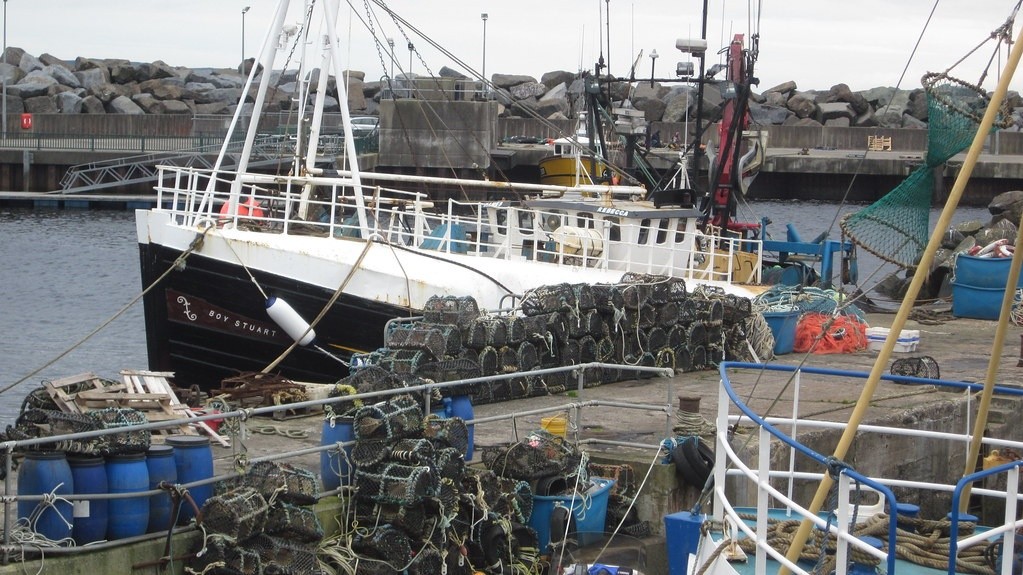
[954,253,1023,288]
[865,326,919,352]
[763,311,798,355]
[951,283,1023,320]
[515,476,614,556]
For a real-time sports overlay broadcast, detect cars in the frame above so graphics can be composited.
[349,117,380,131]
[502,135,549,144]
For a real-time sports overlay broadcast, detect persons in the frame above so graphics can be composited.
[670,132,681,150]
[651,130,663,148]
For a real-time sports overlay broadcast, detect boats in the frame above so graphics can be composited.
[136,0,769,395]
[664,0,1023,575]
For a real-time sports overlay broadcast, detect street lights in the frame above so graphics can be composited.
[241,5,251,130]
[387,38,395,80]
[481,12,488,99]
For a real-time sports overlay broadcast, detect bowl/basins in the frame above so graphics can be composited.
[190,408,223,432]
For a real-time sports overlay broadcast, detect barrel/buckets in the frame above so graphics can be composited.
[420,394,475,461]
[320,416,356,492]
[67,456,108,546]
[105,454,150,542]
[146,445,177,533]
[541,413,568,445]
[163,434,213,526]
[952,252,1022,322]
[17,452,73,548]
